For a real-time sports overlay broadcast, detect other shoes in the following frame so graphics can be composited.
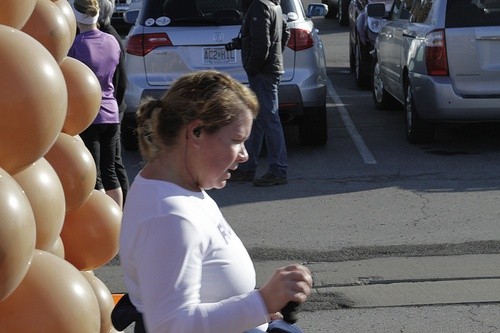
[229,168,255,183]
[253,172,287,187]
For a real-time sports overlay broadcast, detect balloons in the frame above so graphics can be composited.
[0,0,123,333]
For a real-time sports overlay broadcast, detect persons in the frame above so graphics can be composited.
[228,0,291,186]
[67,0,123,212]
[96,0,129,207]
[118,71,313,333]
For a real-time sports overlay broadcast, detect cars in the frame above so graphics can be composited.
[346,0,399,91]
[118,0,329,146]
[367,0,500,145]
[320,0,353,26]
[109,4,131,35]
